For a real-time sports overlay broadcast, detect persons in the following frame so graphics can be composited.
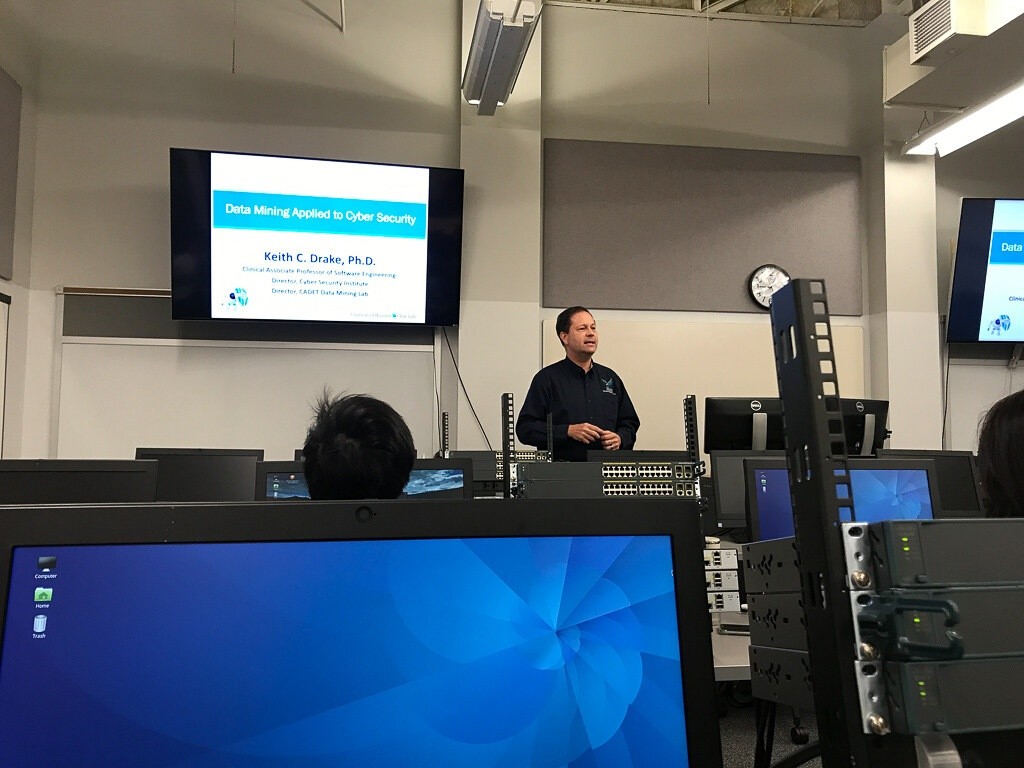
[516,306,641,462]
[977,389,1024,518]
[301,394,416,502]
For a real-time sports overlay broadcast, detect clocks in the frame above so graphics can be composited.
[747,265,791,308]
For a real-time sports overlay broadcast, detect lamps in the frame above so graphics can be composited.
[462,0,545,116]
[901,76,1024,157]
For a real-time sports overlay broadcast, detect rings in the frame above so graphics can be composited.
[610,445,613,450]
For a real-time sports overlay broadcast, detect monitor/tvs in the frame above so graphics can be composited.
[170,147,465,328]
[944,197,1024,343]
[704,397,785,455]
[826,398,890,457]
[0,448,473,504]
[1,498,724,768]
[709,449,986,543]
